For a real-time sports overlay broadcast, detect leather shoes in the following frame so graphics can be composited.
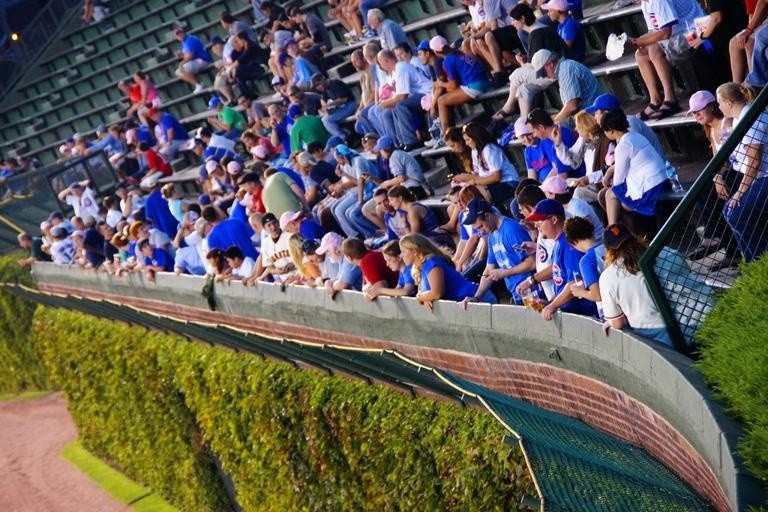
[708,258,741,273]
[396,141,420,153]
[687,240,720,262]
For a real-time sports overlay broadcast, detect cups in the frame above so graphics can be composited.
[517,288,539,311]
[696,225,706,242]
[570,280,585,299]
[683,29,697,45]
[102,254,122,274]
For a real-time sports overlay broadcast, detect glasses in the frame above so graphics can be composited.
[360,136,378,141]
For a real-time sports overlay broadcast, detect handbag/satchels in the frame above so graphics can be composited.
[420,177,436,196]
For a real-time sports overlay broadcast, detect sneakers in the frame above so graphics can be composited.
[346,27,378,47]
[423,137,447,150]
[427,119,440,132]
[493,70,510,88]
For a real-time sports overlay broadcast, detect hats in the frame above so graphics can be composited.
[603,223,632,250]
[540,1,571,12]
[280,209,303,232]
[321,135,342,153]
[284,37,297,49]
[212,36,223,45]
[41,95,276,254]
[531,49,552,79]
[686,91,715,115]
[513,114,534,138]
[372,136,393,153]
[525,199,564,222]
[309,72,326,89]
[315,232,343,256]
[286,85,301,98]
[428,35,448,52]
[410,39,432,50]
[173,28,184,36]
[460,199,491,225]
[586,93,619,113]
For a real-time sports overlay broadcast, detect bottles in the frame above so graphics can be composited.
[663,160,684,195]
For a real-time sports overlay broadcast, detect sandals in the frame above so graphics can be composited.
[651,99,683,122]
[636,101,660,121]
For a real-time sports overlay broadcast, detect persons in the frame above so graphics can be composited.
[0,0,768,350]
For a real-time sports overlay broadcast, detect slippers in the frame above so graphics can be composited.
[430,226,459,236]
[490,108,518,121]
